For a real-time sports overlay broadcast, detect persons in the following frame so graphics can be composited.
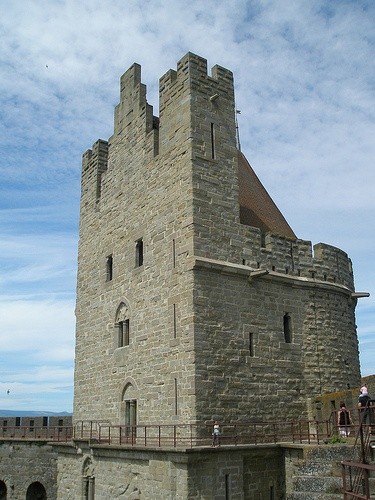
[211,421,221,447]
[358,391,373,436]
[338,403,354,437]
[359,383,368,397]
